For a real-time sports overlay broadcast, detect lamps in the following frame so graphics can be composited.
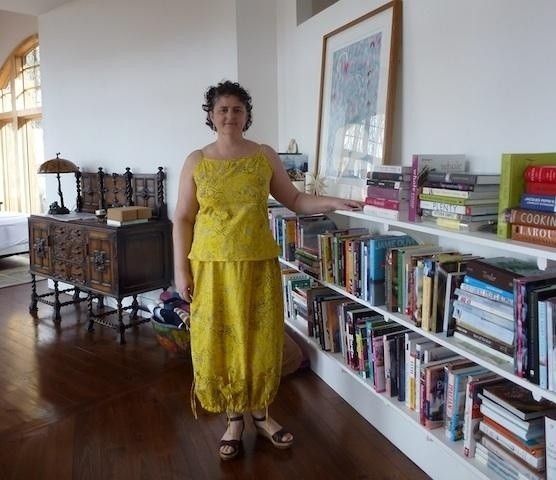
[37,149,81,215]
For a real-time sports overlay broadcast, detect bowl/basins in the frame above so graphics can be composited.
[149,314,190,360]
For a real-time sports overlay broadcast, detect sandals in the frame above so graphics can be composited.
[252,416,294,449]
[219,417,244,460]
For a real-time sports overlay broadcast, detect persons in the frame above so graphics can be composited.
[174,81,367,461]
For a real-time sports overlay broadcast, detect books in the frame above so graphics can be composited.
[364,231,556,391]
[383,319,556,480]
[319,293,368,372]
[263,208,363,301]
[363,153,556,248]
[278,266,338,350]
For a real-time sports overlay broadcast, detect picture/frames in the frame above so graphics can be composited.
[312,0,403,203]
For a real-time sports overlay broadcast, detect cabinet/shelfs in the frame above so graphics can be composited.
[262,257,556,480]
[266,207,556,406]
